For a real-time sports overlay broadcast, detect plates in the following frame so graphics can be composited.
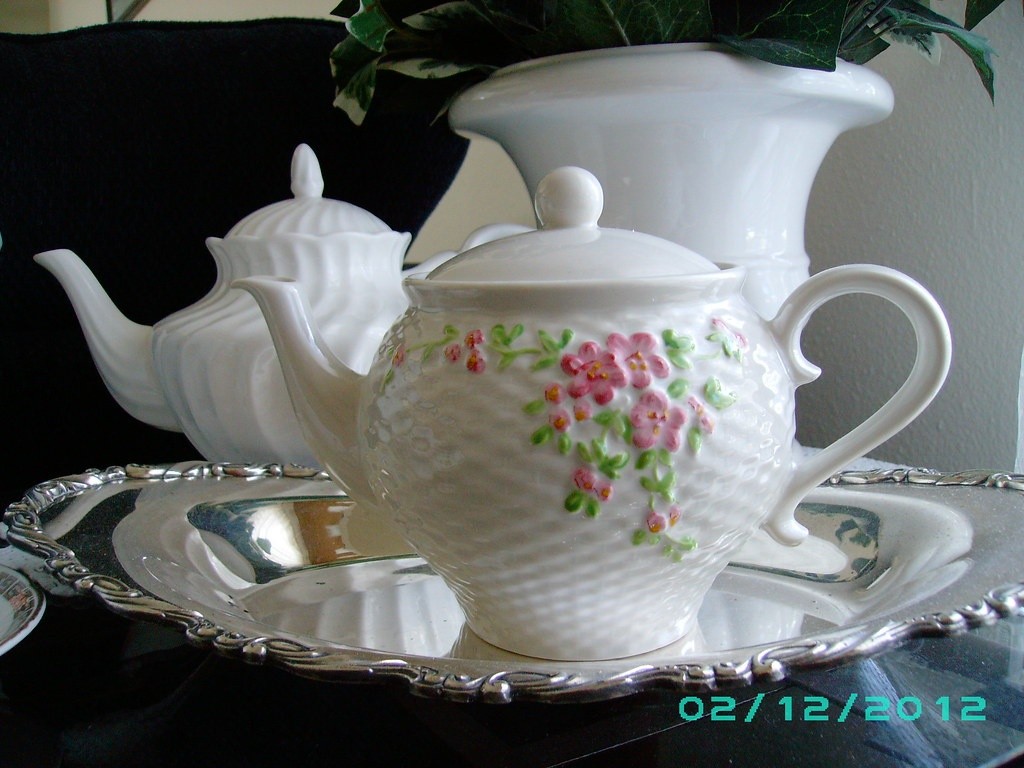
[0,562,48,656]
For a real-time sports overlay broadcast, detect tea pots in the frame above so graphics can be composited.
[33,144,537,464]
[230,167,952,661]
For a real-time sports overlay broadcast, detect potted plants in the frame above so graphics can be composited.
[329,0,1007,323]
